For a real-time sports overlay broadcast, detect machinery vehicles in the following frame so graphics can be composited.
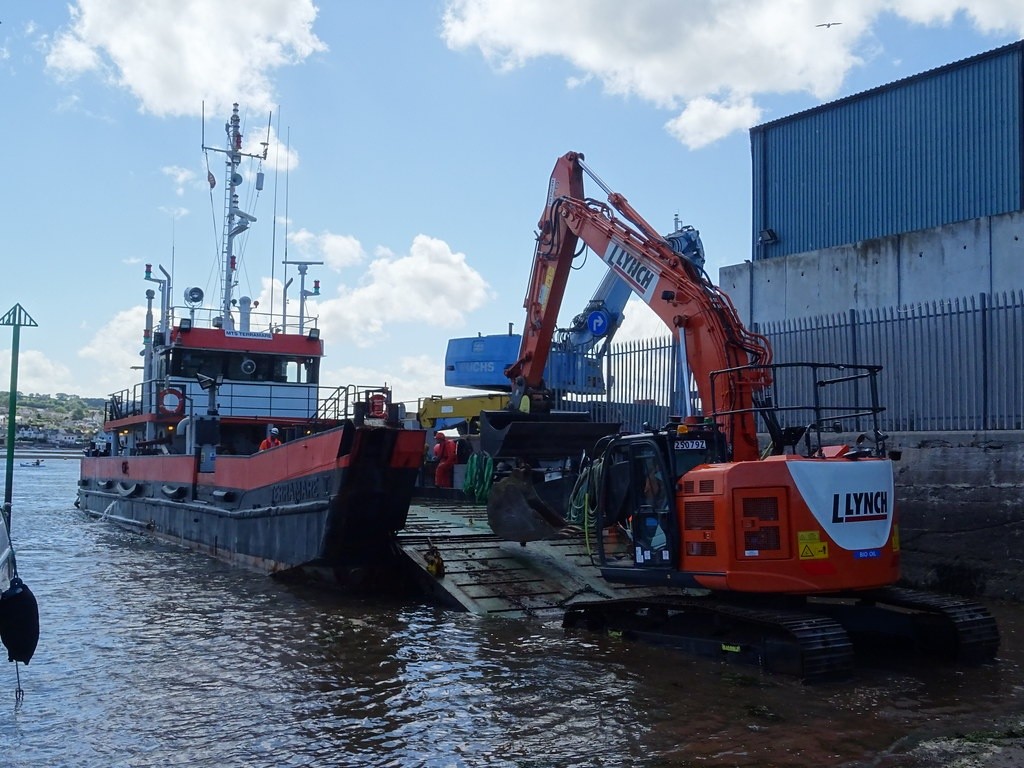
[478,148,1001,688]
[421,210,710,500]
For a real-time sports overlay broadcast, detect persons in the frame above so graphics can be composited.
[258,427,282,452]
[433,432,455,488]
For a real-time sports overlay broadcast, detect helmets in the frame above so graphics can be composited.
[433,433,445,440]
[270,428,279,436]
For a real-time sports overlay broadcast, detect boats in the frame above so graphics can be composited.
[74,93,432,591]
[21,461,46,470]
